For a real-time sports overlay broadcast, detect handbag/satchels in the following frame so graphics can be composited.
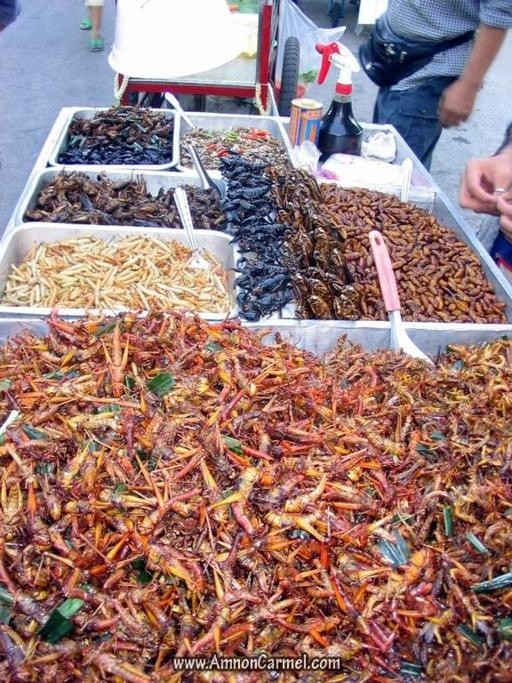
[356,10,436,92]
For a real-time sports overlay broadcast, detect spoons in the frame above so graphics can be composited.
[188,142,224,200]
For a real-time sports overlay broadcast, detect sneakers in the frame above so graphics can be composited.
[89,36,104,52]
[79,15,92,31]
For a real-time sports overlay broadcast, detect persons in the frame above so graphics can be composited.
[357,0,512,173]
[457,117,512,286]
[79,0,106,52]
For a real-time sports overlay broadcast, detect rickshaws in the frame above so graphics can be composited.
[117,0,301,116]
